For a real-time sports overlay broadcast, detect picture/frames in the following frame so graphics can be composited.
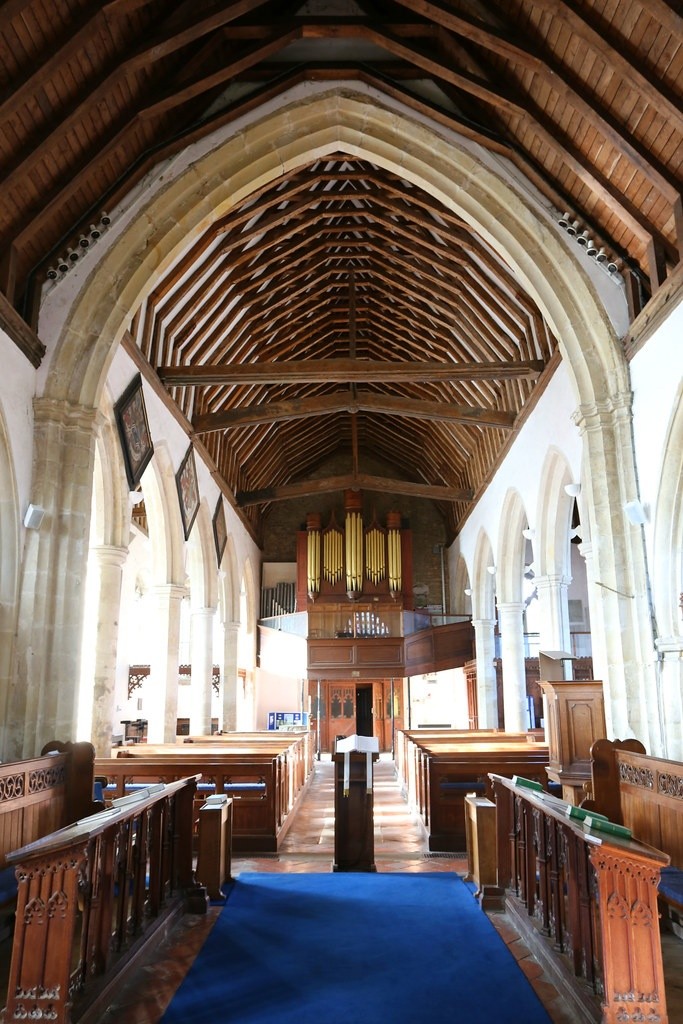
[114,371,155,494]
[175,441,202,541]
[212,492,228,570]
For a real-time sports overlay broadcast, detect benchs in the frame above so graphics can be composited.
[395,728,547,855]
[92,729,317,853]
[655,865,683,939]
[0,865,18,906]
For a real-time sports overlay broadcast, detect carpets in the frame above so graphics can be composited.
[155,870,561,1024]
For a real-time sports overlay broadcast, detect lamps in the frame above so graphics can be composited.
[100,211,111,226]
[130,490,143,506]
[577,229,589,245]
[47,266,58,282]
[597,247,607,262]
[219,570,226,578]
[608,258,623,275]
[566,220,580,235]
[487,564,496,574]
[66,248,79,261]
[239,591,246,597]
[464,588,472,595]
[564,482,580,497]
[558,211,570,227]
[57,257,69,273]
[78,234,90,249]
[621,499,649,526]
[523,526,536,539]
[586,240,600,256]
[22,500,46,531]
[89,224,101,239]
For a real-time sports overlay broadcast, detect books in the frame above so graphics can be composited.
[511,775,543,791]
[565,804,608,821]
[583,815,631,838]
[206,794,227,803]
